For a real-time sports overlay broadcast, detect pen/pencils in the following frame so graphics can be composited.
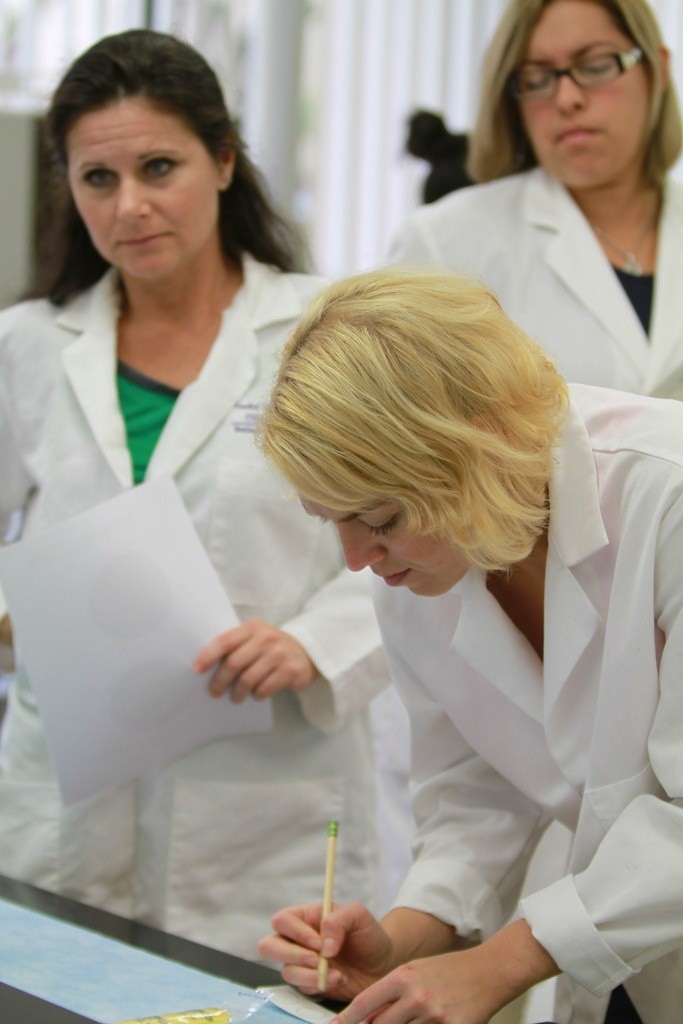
[314,819,339,993]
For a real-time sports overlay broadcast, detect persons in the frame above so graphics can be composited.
[261,271,683,1024]
[406,104,474,206]
[394,1,683,397]
[0,30,391,960]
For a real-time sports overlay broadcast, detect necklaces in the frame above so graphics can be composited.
[570,190,662,277]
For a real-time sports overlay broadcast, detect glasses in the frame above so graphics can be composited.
[512,47,642,100]
